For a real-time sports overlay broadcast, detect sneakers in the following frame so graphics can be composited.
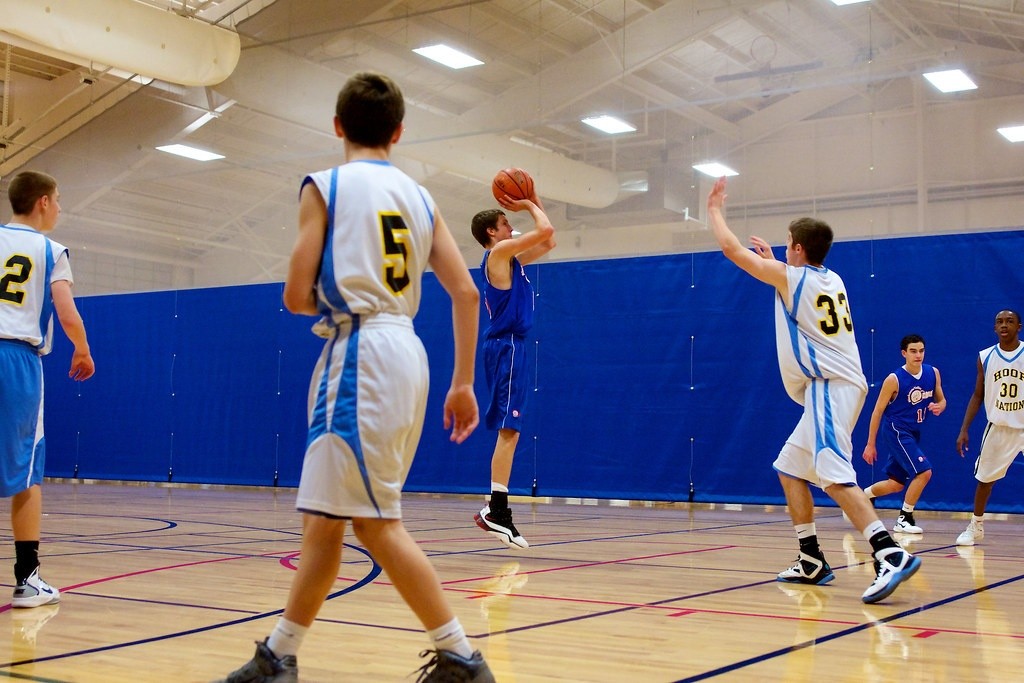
[893,507,923,534]
[777,549,835,584]
[227,636,297,683]
[956,518,984,545]
[406,649,496,683]
[474,501,529,550]
[843,510,854,526]
[11,562,61,608]
[861,542,922,603]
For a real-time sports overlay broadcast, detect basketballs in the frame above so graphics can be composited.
[491,169,532,205]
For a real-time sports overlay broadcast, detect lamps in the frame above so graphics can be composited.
[922,0,979,93]
[411,0,485,70]
[692,52,741,177]
[153,82,227,162]
[579,0,639,135]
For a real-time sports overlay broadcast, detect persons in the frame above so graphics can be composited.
[842,334,947,534]
[482,560,530,682]
[10,605,56,678]
[223,76,496,683]
[955,548,1024,683]
[0,171,96,608]
[956,308,1024,547]
[471,195,556,550]
[843,535,923,609]
[785,585,911,683]
[707,176,922,606]
